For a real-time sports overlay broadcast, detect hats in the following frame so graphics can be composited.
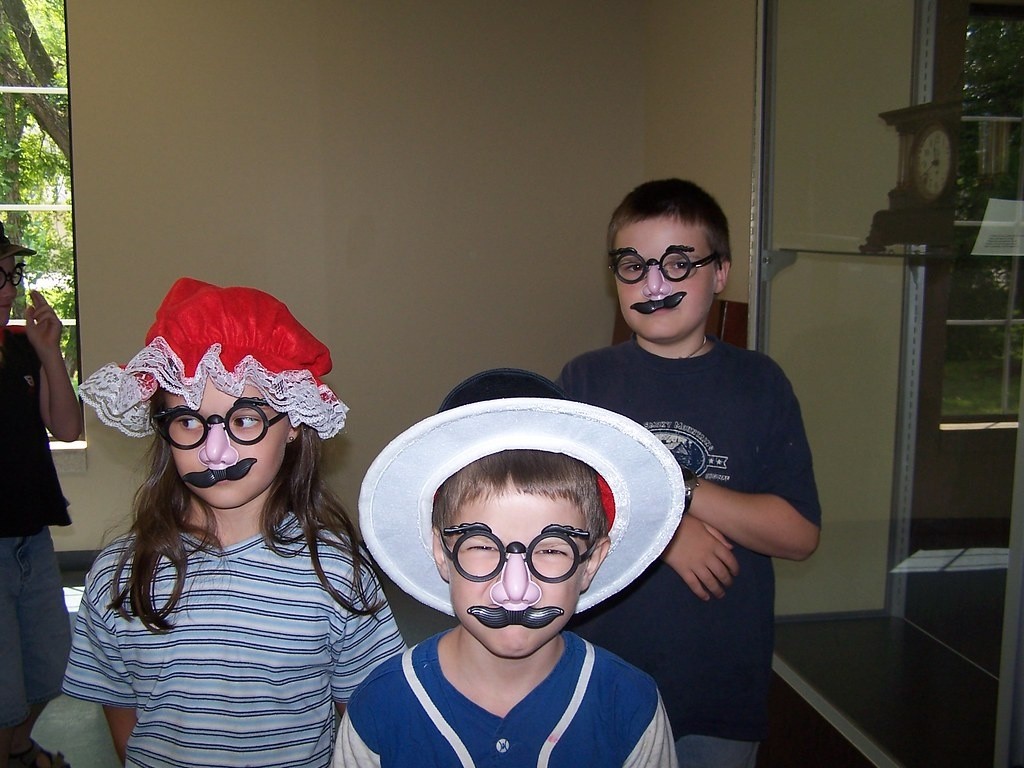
[357,368,685,618]
[0,220,37,261]
[76,278,350,440]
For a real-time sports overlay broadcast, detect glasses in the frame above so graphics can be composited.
[0,262,26,290]
[437,521,600,584]
[608,244,720,285]
[149,397,288,450]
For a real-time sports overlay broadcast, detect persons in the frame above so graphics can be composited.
[0,222,83,768]
[330,368,681,768]
[556,177,822,768]
[62,278,406,768]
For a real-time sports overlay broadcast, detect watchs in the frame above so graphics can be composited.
[683,468,699,514]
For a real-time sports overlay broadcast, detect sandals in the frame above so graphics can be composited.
[7,737,71,768]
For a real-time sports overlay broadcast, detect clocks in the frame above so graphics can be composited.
[859,101,970,254]
